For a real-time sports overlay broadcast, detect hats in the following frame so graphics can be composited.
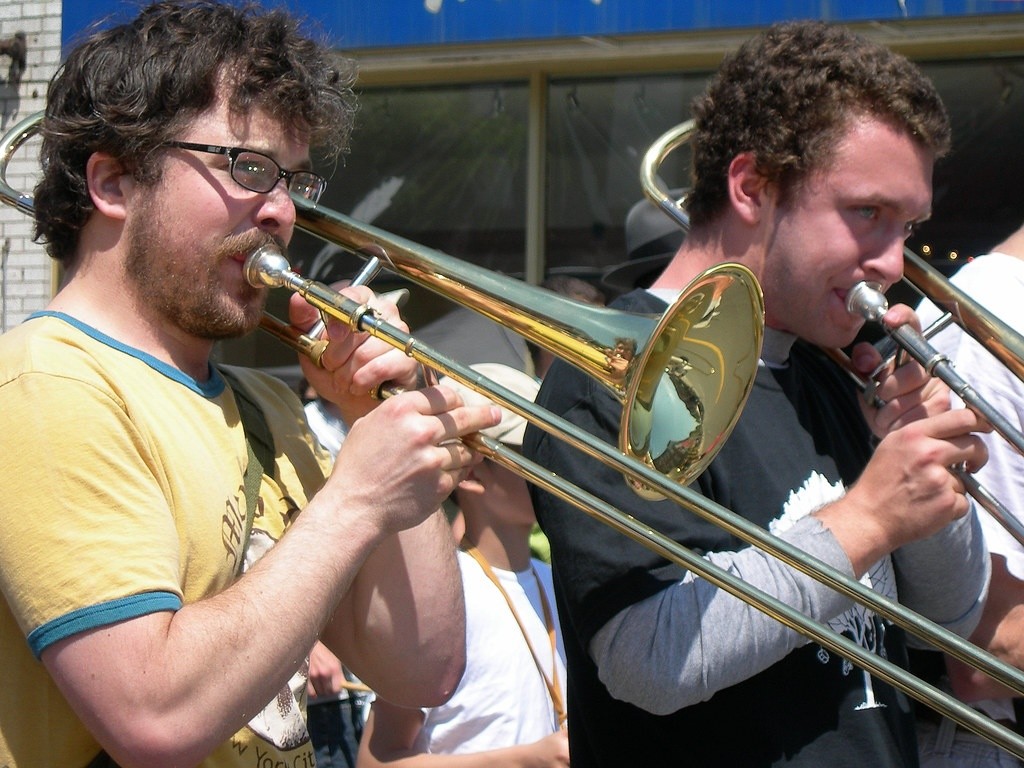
[602,187,697,290]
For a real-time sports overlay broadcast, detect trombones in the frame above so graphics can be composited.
[641,115,1024,548]
[0,109,1024,757]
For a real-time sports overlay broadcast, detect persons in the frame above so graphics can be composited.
[913,223,1024,767]
[597,337,639,390]
[350,362,571,768]
[518,18,994,766]
[298,184,694,457]
[1,1,502,768]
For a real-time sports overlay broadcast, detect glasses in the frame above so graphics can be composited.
[139,140,324,208]
